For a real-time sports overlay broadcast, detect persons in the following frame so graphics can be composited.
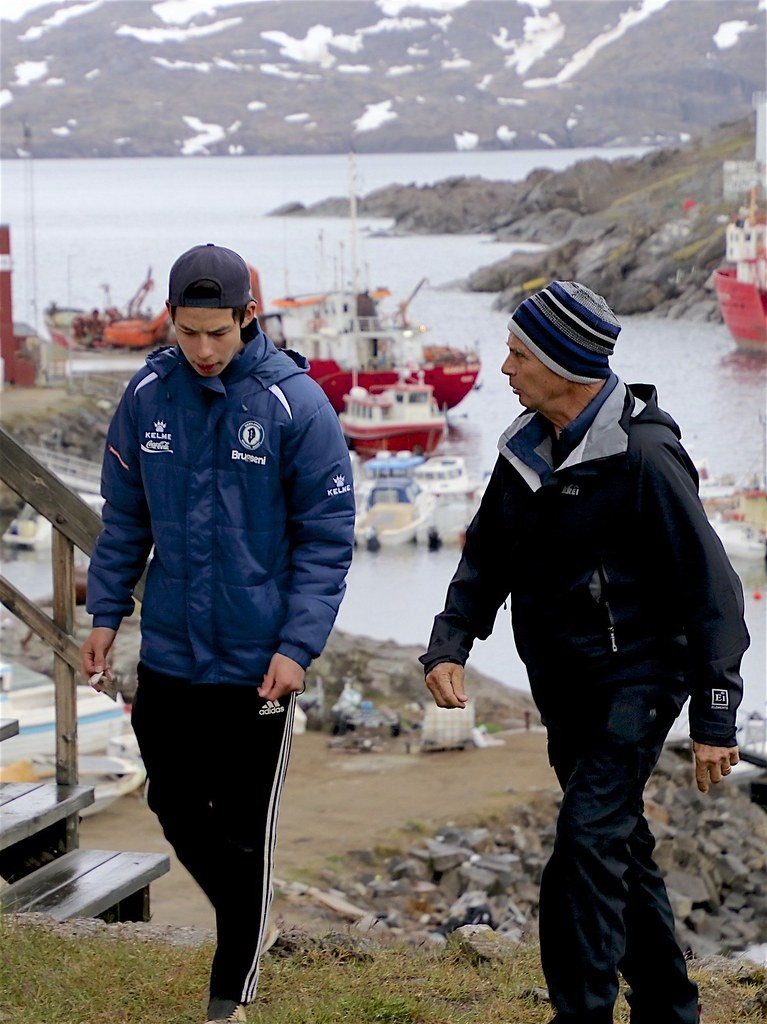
[80,243,354,1023]
[417,279,751,1024]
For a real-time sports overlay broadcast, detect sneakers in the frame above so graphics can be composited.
[200,917,280,1023]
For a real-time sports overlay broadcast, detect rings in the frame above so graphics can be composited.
[722,767,731,771]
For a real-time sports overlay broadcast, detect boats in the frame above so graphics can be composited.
[711,183,767,358]
[340,370,448,456]
[262,180,481,418]
[356,457,471,550]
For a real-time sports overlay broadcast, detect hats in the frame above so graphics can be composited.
[169,244,259,308]
[507,278,624,384]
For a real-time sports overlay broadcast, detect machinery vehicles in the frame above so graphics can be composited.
[103,266,262,352]
